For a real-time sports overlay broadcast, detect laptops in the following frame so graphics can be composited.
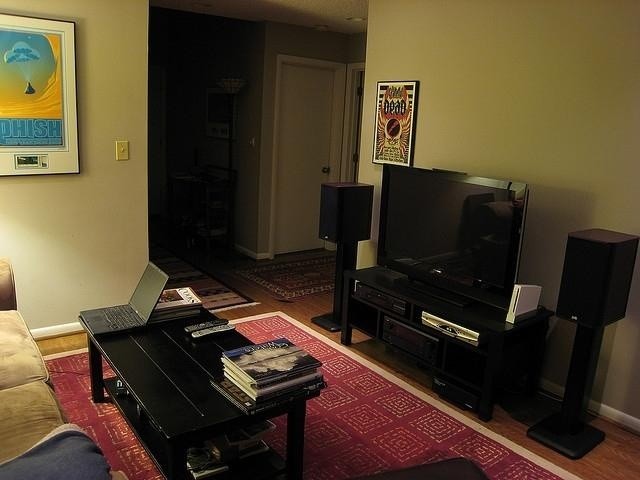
[80,261,170,338]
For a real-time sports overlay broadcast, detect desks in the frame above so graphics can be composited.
[341,266,556,423]
[168,172,233,257]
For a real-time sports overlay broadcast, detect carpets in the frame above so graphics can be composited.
[148,239,261,314]
[42,310,583,480]
[221,251,338,304]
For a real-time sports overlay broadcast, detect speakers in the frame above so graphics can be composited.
[556,226,639,328]
[318,182,374,243]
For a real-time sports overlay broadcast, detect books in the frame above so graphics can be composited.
[148,287,202,325]
[209,338,327,415]
[186,420,277,480]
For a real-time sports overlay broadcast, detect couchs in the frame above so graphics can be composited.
[0,260,126,480]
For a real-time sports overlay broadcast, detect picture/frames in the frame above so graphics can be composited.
[372,80,420,167]
[0,12,79,178]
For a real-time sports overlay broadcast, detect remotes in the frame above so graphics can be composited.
[184,318,228,333]
[191,325,236,339]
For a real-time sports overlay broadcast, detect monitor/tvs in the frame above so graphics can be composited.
[377,163,530,311]
